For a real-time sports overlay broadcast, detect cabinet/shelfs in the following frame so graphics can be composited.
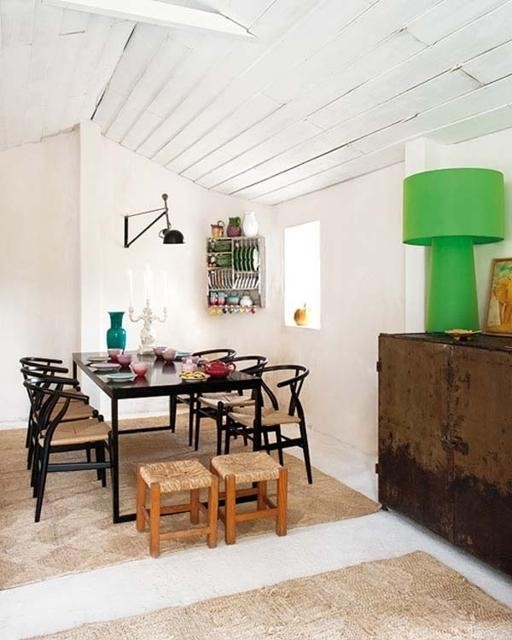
[374,326,511,582]
[204,233,266,313]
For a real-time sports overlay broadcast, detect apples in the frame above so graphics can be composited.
[295,308,310,326]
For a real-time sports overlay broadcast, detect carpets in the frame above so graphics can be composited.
[1,411,387,592]
[20,544,511,638]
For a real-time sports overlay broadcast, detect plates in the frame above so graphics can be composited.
[85,357,111,363]
[445,332,481,341]
[177,352,192,360]
[93,363,120,371]
[181,375,210,384]
[104,372,138,382]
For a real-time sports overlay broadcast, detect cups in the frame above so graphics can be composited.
[211,224,223,238]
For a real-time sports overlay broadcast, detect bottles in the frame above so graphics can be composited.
[107,312,126,350]
[210,292,225,306]
[295,307,308,325]
[226,289,253,306]
[227,217,242,236]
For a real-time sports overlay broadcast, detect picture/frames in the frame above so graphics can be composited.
[478,255,512,340]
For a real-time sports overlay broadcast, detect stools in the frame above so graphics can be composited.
[130,456,220,560]
[208,448,293,547]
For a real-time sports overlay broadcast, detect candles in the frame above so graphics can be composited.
[128,286,134,309]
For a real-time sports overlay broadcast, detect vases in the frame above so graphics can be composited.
[241,211,258,237]
[105,310,127,354]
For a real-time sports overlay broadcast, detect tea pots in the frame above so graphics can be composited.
[204,360,236,378]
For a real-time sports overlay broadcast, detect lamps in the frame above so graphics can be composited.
[401,166,506,333]
[123,192,186,249]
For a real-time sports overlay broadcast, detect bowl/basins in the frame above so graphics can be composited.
[108,348,137,364]
[131,364,150,376]
[153,346,177,362]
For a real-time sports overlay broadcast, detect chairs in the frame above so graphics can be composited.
[165,347,315,492]
[18,352,116,523]
